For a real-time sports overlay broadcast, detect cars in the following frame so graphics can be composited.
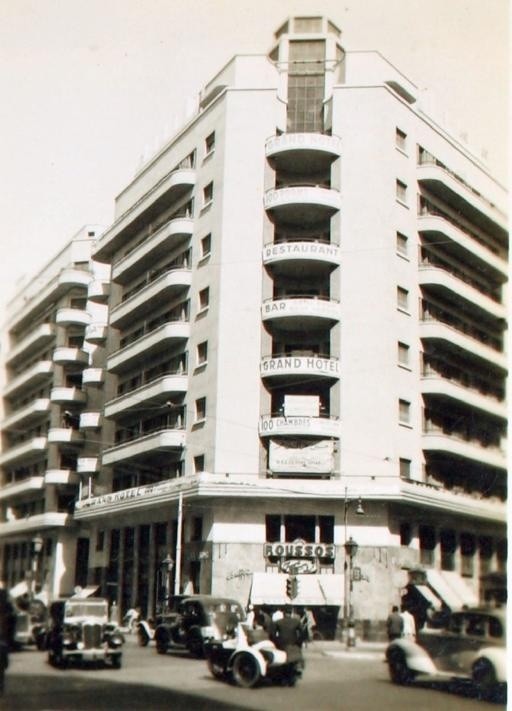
[40,596,125,666]
[387,606,506,702]
[136,593,245,655]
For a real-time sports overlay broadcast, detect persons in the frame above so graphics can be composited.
[397,603,416,638]
[271,604,309,665]
[122,607,140,633]
[382,605,403,662]
[246,613,271,646]
[299,607,318,641]
[271,606,284,622]
[1,588,19,697]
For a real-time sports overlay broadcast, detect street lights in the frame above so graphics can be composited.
[161,556,174,612]
[345,537,359,647]
[25,533,44,596]
